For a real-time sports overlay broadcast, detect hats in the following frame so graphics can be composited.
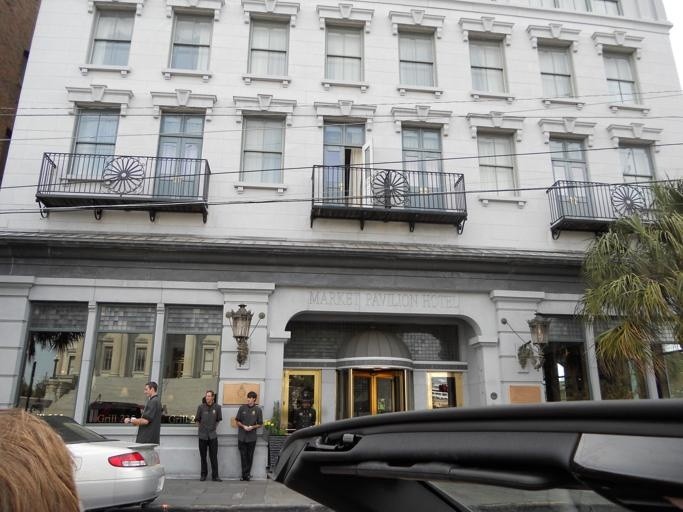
[299,391,313,402]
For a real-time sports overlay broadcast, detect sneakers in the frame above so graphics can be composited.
[240,475,251,481]
[212,477,223,482]
[200,471,208,481]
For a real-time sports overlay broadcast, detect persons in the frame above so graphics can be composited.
[122,380,162,446]
[192,389,225,482]
[0,402,81,511]
[291,395,317,432]
[232,391,265,482]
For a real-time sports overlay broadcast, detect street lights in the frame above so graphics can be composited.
[50,354,61,380]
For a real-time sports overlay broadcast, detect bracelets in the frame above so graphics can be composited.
[127,417,132,423]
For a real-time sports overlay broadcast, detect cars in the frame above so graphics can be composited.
[29,413,167,511]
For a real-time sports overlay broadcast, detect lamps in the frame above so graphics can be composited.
[225,303,265,370]
[501,312,552,373]
